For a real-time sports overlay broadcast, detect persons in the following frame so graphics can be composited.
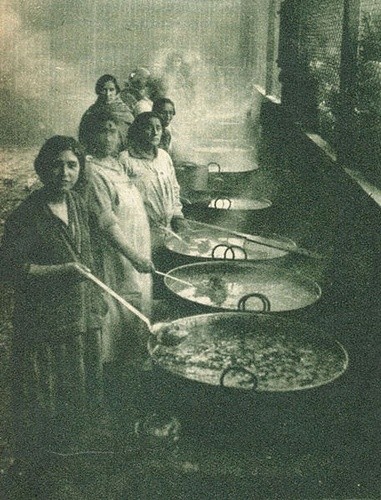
[74,107,162,417]
[112,110,196,259]
[120,87,155,116]
[9,131,140,468]
[79,72,142,157]
[151,97,175,155]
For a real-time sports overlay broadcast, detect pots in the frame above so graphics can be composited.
[146,295,351,403]
[181,156,258,194]
[165,228,298,268]
[181,178,272,235]
[164,245,323,314]
